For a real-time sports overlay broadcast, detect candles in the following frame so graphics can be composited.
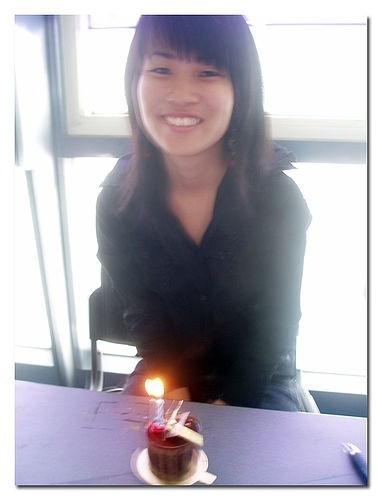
[144,376,165,428]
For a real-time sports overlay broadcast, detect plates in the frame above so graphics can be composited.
[130,446,218,486]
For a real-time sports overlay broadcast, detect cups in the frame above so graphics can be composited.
[145,413,202,481]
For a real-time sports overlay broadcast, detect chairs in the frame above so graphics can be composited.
[88,287,321,414]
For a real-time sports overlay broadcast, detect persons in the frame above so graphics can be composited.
[88,15,314,413]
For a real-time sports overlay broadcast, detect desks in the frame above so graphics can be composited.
[14,380,367,486]
